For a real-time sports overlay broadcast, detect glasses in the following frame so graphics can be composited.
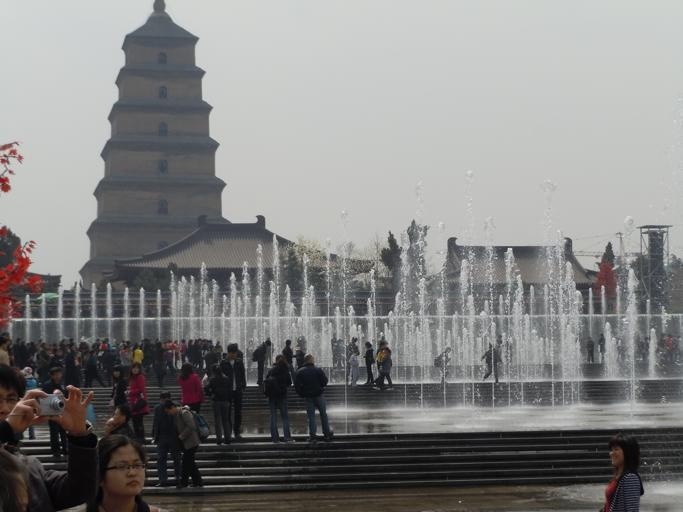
[103,462,146,472]
[0,396,18,404]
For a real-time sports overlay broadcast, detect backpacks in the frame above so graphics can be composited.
[178,408,209,439]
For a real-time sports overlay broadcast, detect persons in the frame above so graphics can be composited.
[599,434,644,512]
[434,347,451,387]
[598,333,683,365]
[586,335,594,364]
[480,336,512,386]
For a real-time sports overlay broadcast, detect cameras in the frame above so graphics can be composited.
[36,394,65,416]
